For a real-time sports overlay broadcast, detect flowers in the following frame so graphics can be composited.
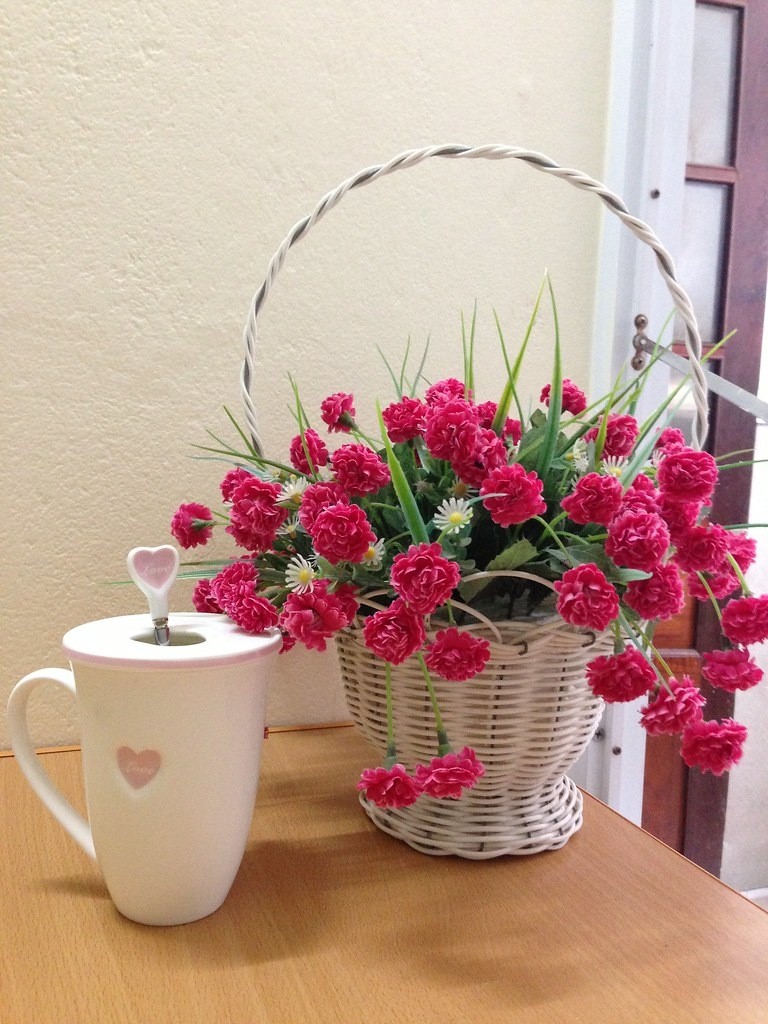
[105,328,768,808]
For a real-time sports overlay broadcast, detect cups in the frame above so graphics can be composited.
[8,612,283,926]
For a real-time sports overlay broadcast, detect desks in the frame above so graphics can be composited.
[0,729,768,1024]
